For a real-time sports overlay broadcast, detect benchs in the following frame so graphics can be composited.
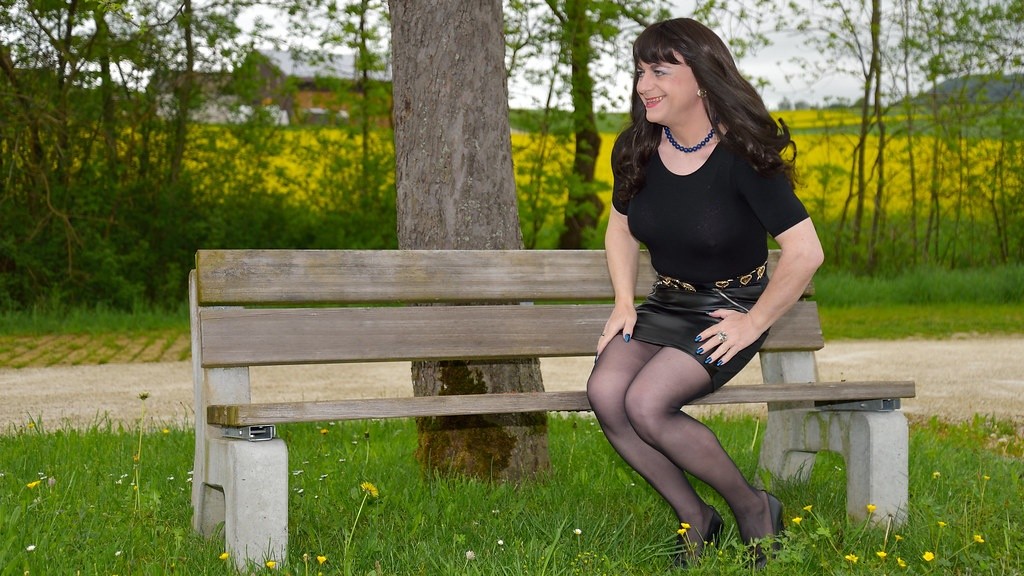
[186,248,915,574]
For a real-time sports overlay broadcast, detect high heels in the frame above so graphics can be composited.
[743,488,788,570]
[672,504,723,569]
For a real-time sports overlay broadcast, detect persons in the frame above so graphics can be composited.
[585,18,825,572]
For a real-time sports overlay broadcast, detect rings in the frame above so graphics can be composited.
[717,330,728,343]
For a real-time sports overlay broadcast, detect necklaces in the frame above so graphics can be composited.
[663,121,719,153]
